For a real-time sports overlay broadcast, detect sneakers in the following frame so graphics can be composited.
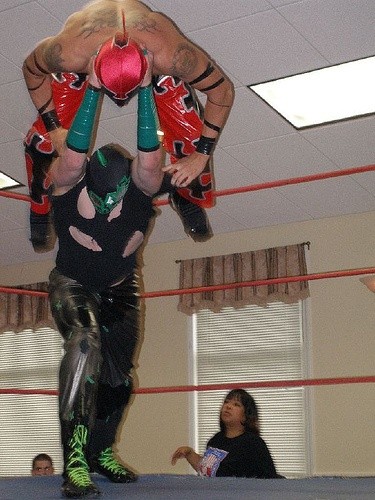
[62,466,101,500]
[85,446,136,483]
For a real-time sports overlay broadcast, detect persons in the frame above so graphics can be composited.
[23,0,236,252]
[46,38,168,500]
[31,454,55,477]
[172,388,277,479]
